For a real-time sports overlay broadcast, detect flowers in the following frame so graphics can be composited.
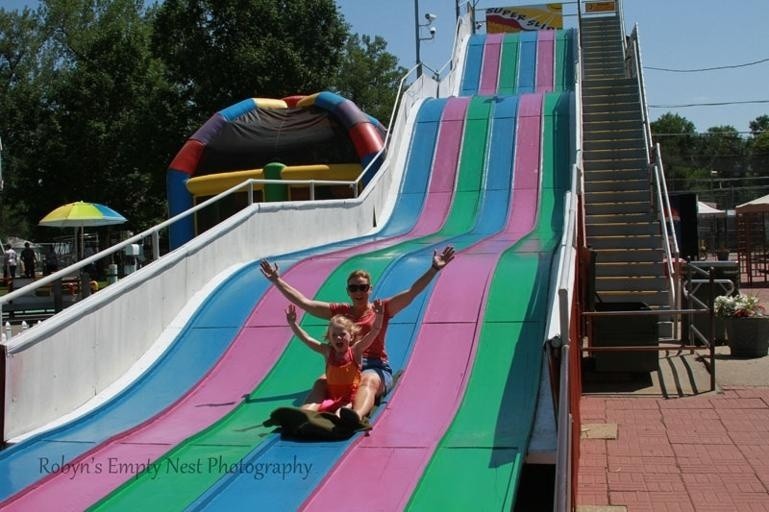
[713,291,765,318]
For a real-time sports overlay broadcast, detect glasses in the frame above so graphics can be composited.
[349,284,370,292]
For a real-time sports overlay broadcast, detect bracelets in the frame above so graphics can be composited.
[431,264,442,271]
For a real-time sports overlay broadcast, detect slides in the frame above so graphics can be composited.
[0,13,581,512]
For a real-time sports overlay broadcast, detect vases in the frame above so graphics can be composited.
[727,317,769,358]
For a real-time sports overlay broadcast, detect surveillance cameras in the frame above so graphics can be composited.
[424,12,438,21]
[430,27,437,34]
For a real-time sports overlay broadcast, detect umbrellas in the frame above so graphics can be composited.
[37,199,129,259]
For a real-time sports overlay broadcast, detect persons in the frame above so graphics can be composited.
[284,297,387,422]
[44,244,58,276]
[3,243,18,278]
[716,241,730,261]
[259,243,457,430]
[19,242,38,277]
